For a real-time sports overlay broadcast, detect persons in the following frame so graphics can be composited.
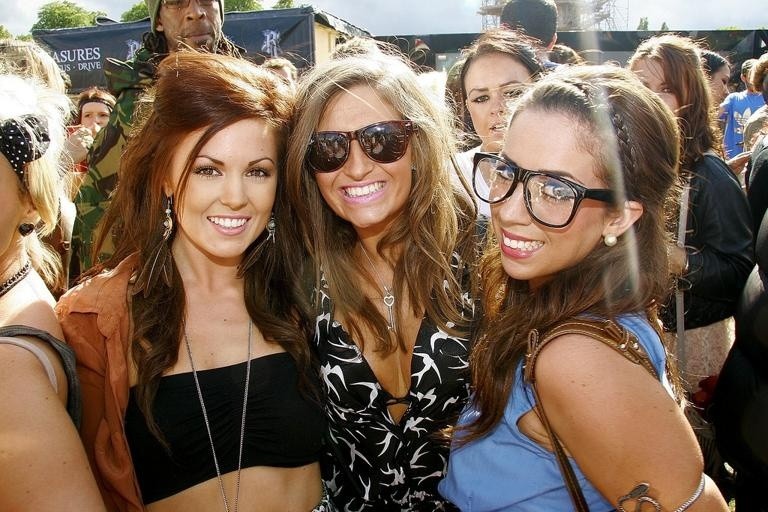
[0,0,768,390]
[0,37,106,505]
[56,50,327,507]
[437,64,737,509]
[241,44,489,508]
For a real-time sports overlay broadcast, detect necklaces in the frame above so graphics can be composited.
[174,305,257,508]
[1,257,34,301]
[356,237,411,332]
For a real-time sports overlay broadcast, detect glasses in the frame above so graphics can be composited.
[307,120,418,173]
[472,151,615,228]
[160,0,217,9]
